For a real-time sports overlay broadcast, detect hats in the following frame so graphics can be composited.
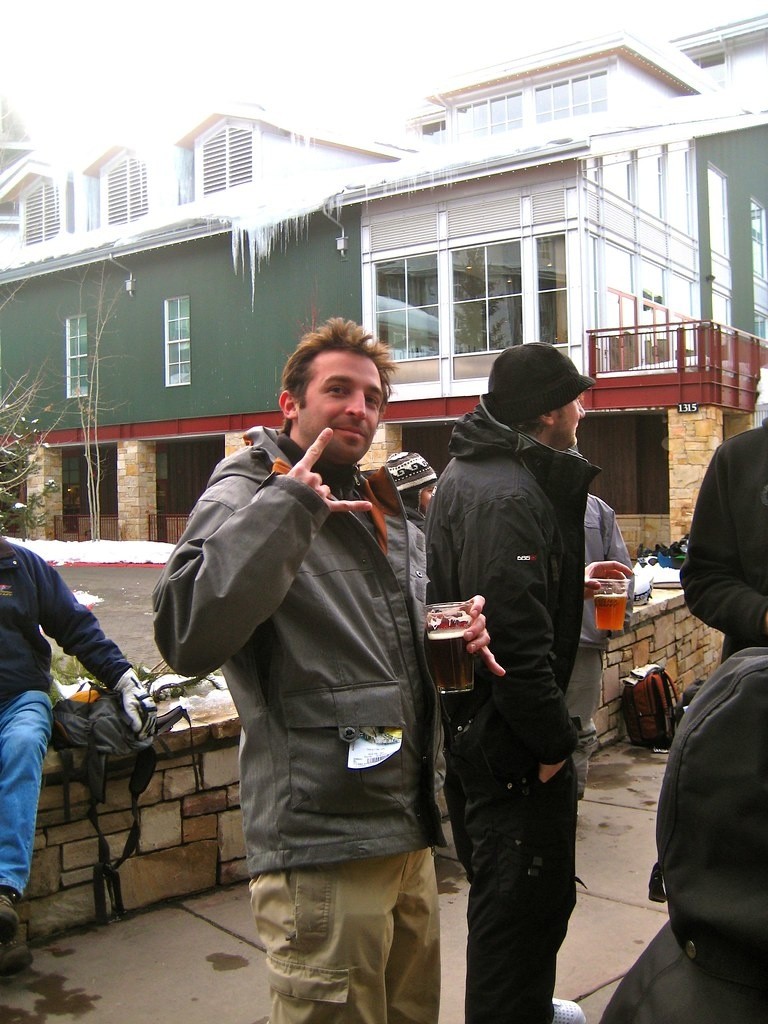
[487,342,595,424]
[384,451,437,511]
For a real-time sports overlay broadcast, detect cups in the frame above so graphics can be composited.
[426,603,475,693]
[592,579,631,631]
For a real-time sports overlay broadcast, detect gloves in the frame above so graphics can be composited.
[114,667,158,741]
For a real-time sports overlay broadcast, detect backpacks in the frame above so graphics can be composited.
[620,663,685,753]
[675,678,706,728]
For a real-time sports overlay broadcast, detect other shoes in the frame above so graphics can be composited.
[551,998,586,1024]
[0,890,22,944]
[577,793,584,800]
[0,940,33,977]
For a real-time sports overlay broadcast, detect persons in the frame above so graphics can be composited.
[152,317,506,1023]
[678,418,768,666]
[567,491,634,801]
[0,531,157,940]
[386,452,438,644]
[421,342,602,1024]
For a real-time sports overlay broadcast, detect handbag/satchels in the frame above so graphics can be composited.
[50,681,185,760]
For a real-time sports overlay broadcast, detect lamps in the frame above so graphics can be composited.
[705,275,715,281]
